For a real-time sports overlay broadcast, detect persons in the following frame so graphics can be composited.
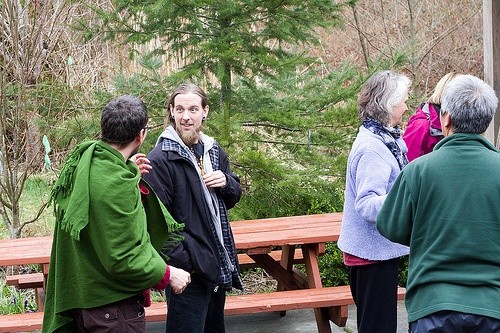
[41,94,192,333]
[401,73,463,162]
[377,74,500,333]
[141,82,245,333]
[337,70,410,333]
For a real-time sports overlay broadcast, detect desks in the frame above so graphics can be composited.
[0,212,343,333]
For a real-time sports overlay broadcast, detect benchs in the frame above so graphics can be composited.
[0,249,405,333]
